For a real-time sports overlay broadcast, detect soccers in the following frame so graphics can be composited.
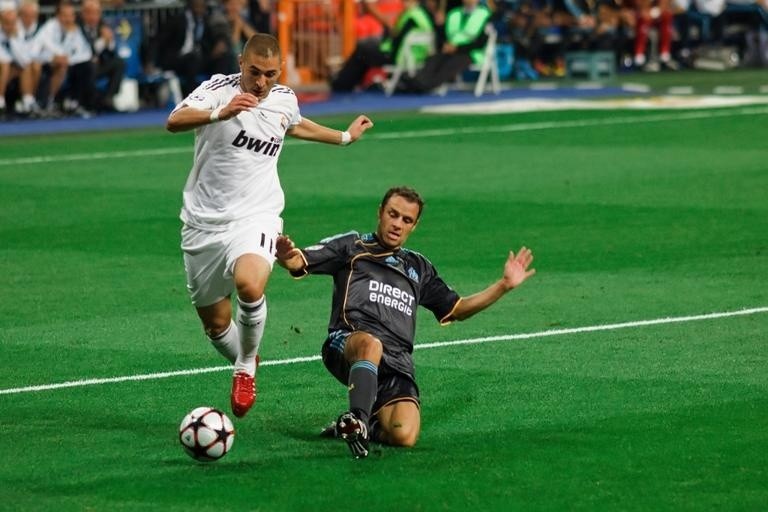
[181,406,234,462]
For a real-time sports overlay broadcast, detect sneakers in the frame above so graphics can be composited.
[321,421,342,438]
[231,372,257,417]
[337,413,369,457]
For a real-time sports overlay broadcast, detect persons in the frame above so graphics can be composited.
[275,186,537,461]
[0,0,271,123]
[326,1,768,98]
[166,33,374,418]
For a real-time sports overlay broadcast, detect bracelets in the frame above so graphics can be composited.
[340,130,353,147]
[210,104,224,122]
